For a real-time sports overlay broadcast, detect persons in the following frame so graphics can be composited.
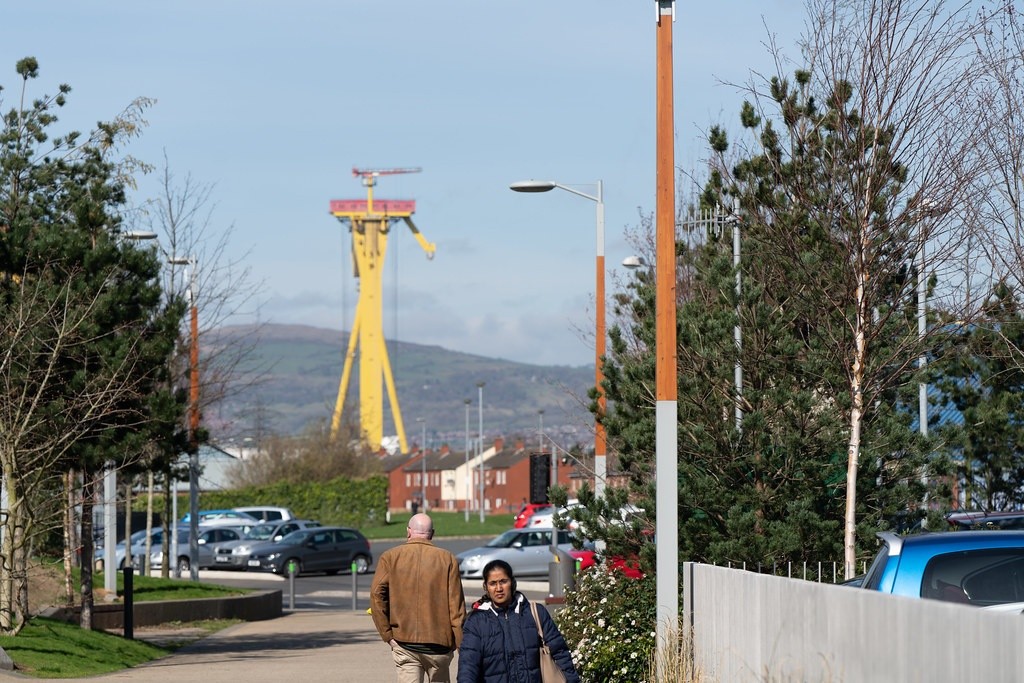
[368,512,466,683]
[456,560,582,683]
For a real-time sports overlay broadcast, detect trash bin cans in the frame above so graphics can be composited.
[547,543,576,598]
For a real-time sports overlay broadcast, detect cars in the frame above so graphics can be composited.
[452,527,596,580]
[511,500,645,533]
[565,528,656,579]
[92,505,294,572]
[831,509,1024,608]
[210,517,327,568]
[245,526,374,578]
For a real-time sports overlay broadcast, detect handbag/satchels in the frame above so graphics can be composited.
[530,601,566,683]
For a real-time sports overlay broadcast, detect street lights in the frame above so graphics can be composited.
[537,409,545,454]
[166,256,201,582]
[98,229,158,602]
[474,378,488,525]
[913,197,949,532]
[416,417,427,516]
[462,397,474,522]
[507,178,609,631]
